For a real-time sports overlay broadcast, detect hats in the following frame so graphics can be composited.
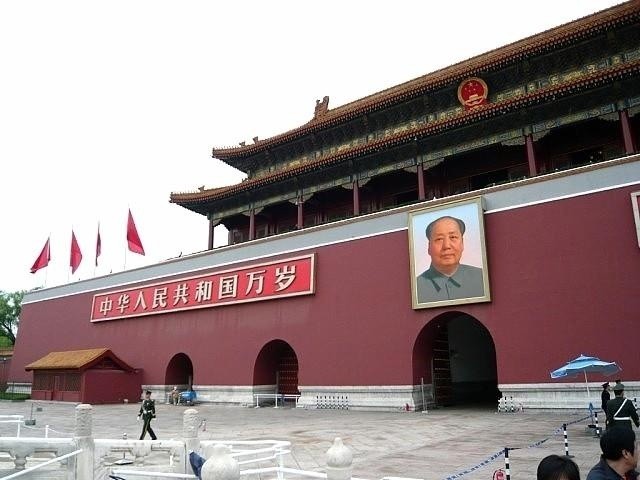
[612,383,625,391]
[601,382,610,388]
[145,389,152,394]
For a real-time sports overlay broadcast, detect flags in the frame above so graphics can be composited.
[127,209,146,256]
[96,233,101,267]
[69,231,82,275]
[30,240,50,274]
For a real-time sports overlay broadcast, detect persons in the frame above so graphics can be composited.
[616,379,621,384]
[137,390,157,440]
[601,382,610,419]
[417,216,484,303]
[537,454,580,480]
[586,428,640,480]
[171,386,179,405]
[606,383,640,430]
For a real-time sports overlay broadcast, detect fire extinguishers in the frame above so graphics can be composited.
[493,468,505,480]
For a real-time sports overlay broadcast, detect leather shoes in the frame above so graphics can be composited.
[152,437,157,440]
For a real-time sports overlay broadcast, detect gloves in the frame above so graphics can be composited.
[137,416,140,420]
[153,418,156,420]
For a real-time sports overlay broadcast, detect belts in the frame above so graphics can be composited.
[613,417,631,420]
[143,411,151,414]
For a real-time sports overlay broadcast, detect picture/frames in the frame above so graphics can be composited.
[405,195,493,311]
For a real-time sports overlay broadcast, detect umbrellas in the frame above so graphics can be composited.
[551,354,622,424]
